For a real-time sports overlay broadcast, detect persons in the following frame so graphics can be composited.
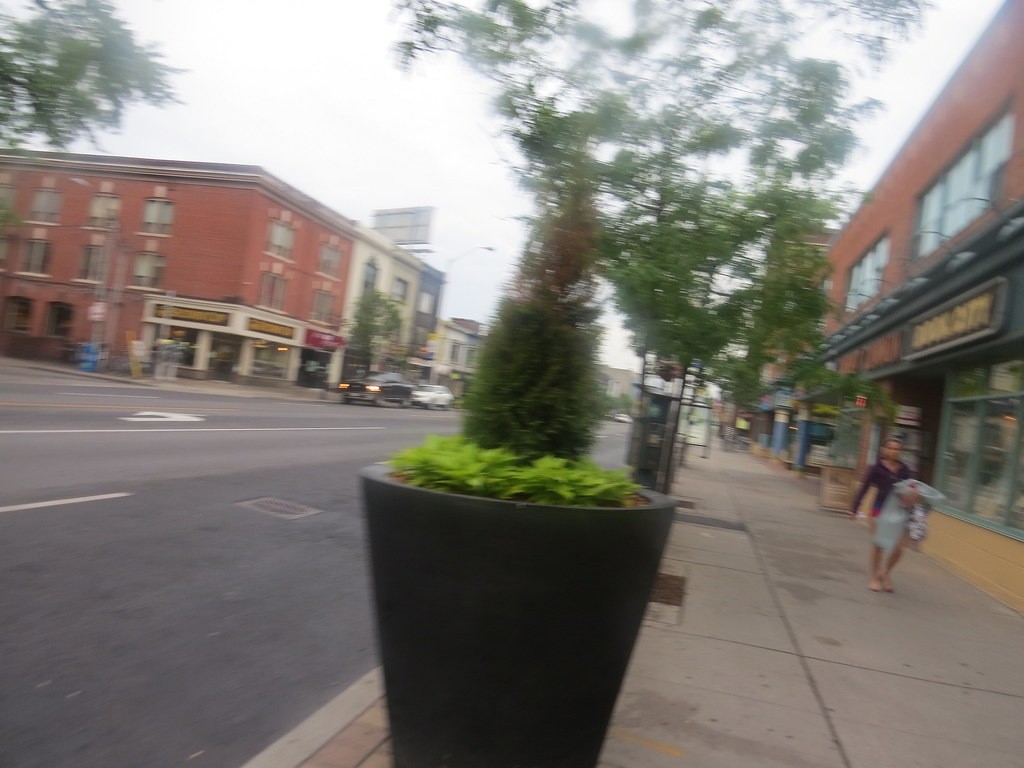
[850,436,922,592]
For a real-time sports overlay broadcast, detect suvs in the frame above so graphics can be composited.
[339,370,415,409]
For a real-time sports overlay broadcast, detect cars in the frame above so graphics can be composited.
[409,384,456,412]
[614,412,633,424]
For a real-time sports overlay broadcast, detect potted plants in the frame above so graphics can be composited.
[358,436,677,768]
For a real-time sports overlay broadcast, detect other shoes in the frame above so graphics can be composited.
[880,576,896,593]
[870,576,882,591]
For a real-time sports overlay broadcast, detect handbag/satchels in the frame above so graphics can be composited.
[908,500,927,541]
